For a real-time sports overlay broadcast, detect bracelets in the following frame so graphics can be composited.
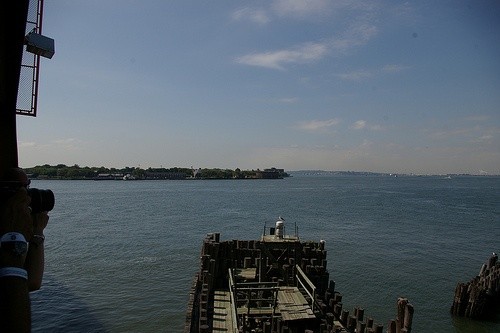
[30,233,45,241]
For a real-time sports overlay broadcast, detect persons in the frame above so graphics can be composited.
[0,181,50,290]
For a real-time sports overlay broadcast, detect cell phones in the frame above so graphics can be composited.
[31,235,43,248]
